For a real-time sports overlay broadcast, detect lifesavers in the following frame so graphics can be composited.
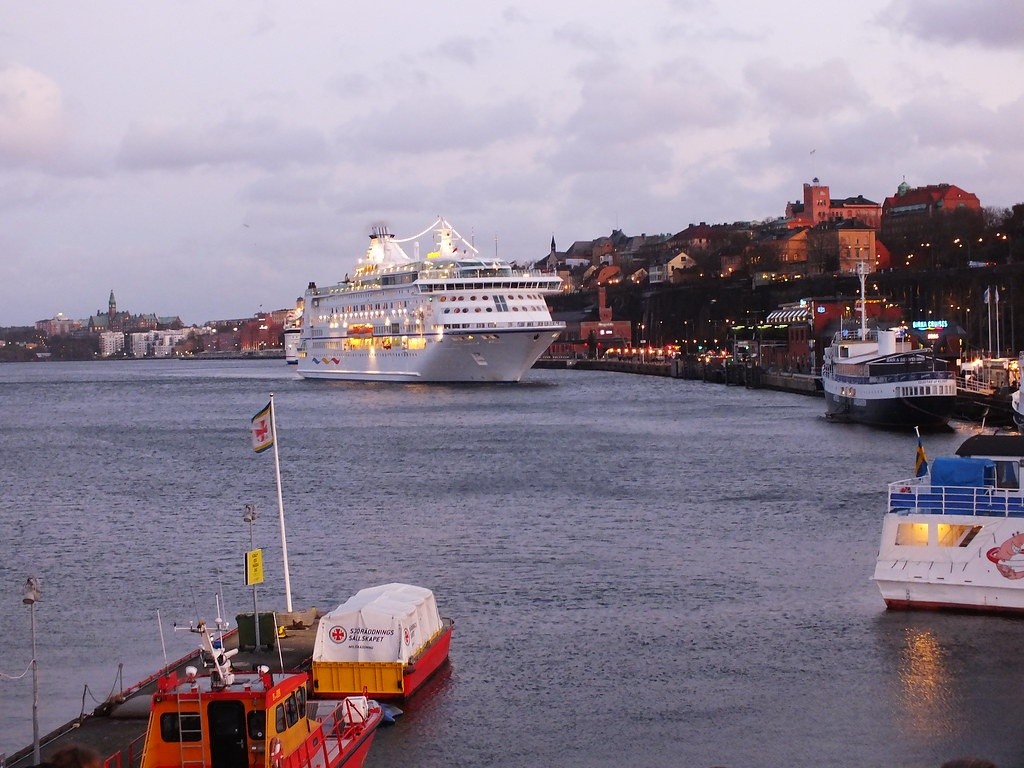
[270,737,284,768]
[901,486,912,493]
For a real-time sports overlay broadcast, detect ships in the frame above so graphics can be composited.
[296,209,568,385]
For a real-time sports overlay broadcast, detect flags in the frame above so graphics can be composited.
[914,437,928,478]
[250,401,274,453]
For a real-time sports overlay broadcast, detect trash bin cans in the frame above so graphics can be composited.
[236,613,275,651]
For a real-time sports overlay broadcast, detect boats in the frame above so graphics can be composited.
[310,582,456,706]
[282,306,304,365]
[867,341,1024,619]
[821,259,958,434]
[139,565,386,768]
